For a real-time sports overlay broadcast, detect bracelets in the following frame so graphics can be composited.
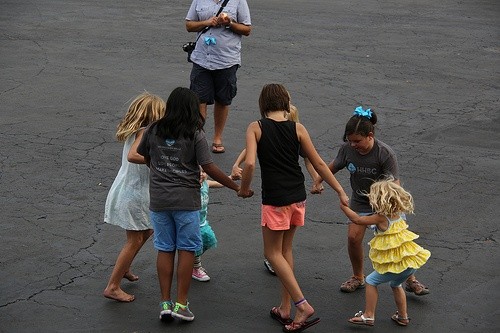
[225,20,233,28]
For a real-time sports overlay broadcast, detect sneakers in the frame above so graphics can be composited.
[192,266,210,281]
[404,275,430,296]
[159,298,194,323]
[340,273,365,292]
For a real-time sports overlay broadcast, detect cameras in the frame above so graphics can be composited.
[182,42,195,52]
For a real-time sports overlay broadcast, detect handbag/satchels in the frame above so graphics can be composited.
[182,41,196,62]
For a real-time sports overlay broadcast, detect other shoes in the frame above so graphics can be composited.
[264,258,275,272]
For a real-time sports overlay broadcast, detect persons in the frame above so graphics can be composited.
[103,83,431,333]
[185,0,252,154]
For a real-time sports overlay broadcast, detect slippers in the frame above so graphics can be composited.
[282,317,321,332]
[212,142,225,153]
[270,306,294,325]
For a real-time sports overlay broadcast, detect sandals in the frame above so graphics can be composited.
[348,310,375,325]
[392,311,411,325]
